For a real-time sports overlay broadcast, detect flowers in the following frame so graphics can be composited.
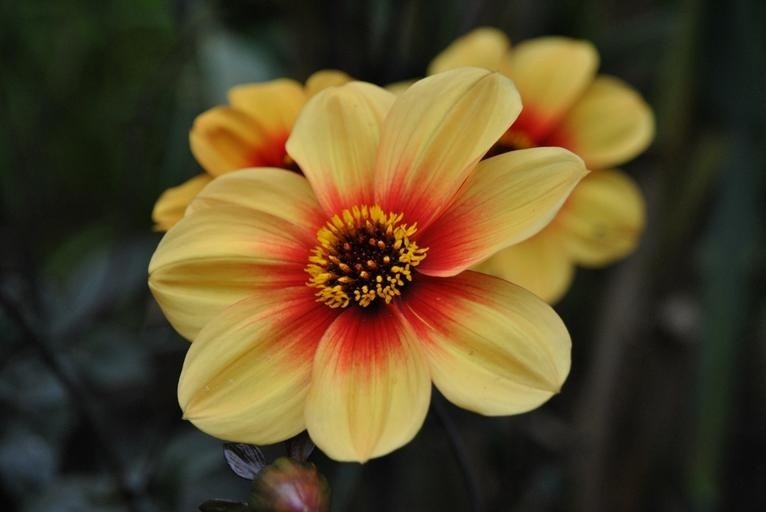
[146,26,658,465]
